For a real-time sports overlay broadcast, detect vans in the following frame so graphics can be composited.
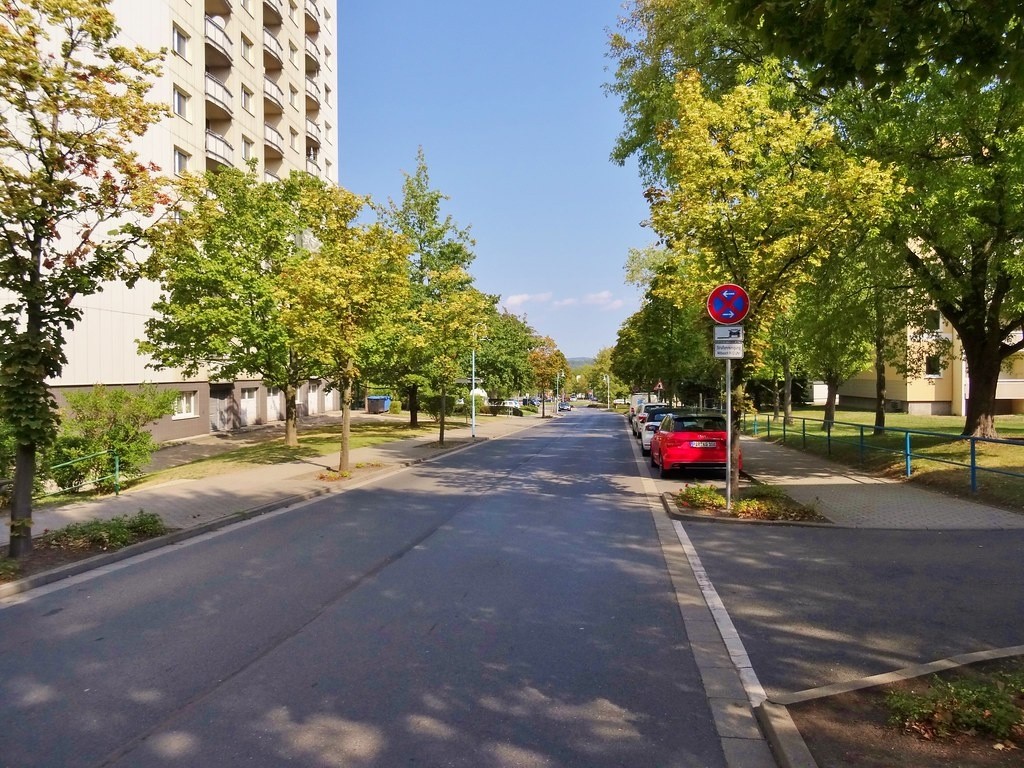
[629,393,658,424]
[523,399,540,408]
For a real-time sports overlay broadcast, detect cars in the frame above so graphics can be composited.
[647,410,742,479]
[557,402,572,412]
[570,396,577,402]
[640,408,694,457]
[631,403,672,439]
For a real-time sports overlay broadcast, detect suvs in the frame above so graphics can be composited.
[493,401,520,409]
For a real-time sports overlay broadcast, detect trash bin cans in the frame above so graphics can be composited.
[368,395,390,414]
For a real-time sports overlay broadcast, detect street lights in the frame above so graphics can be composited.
[471,322,489,440]
[556,369,565,416]
[603,374,610,409]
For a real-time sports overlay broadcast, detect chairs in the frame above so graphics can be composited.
[703,421,717,430]
[674,421,684,430]
[656,414,662,420]
